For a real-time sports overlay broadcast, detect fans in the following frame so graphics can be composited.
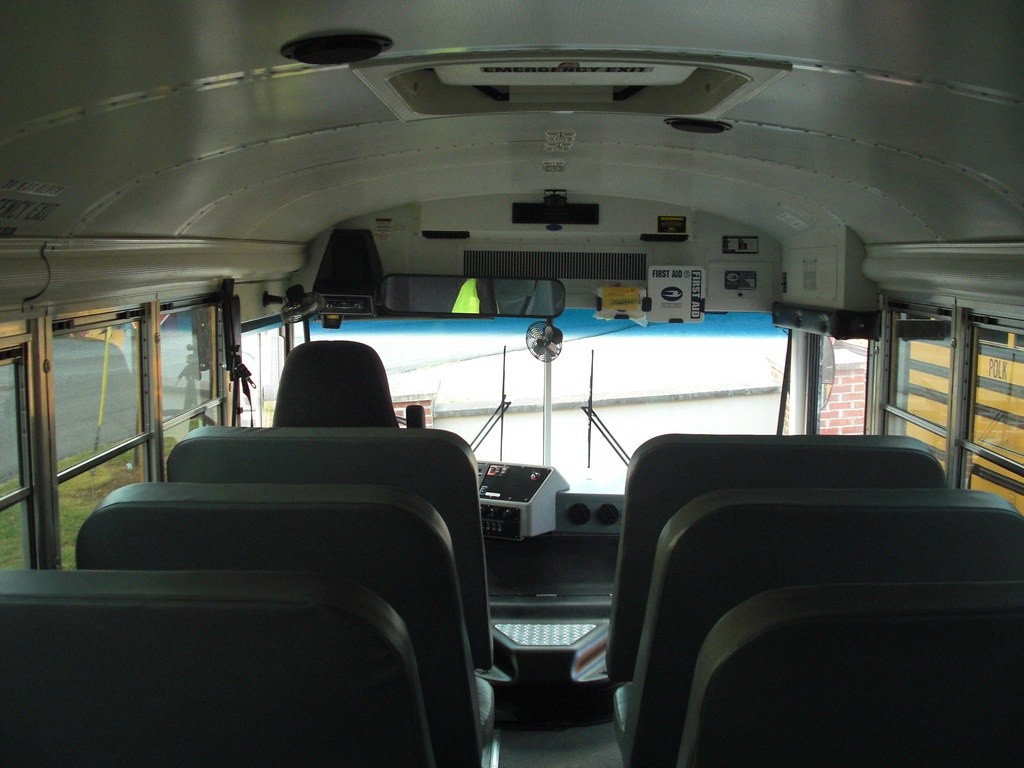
[523,317,565,364]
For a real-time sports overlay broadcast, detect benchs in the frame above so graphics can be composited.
[613,487,1024,767]
[164,426,506,671]
[0,570,442,768]
[70,485,499,756]
[670,576,1023,767]
[607,433,949,685]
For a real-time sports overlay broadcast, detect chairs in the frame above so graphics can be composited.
[272,340,400,427]
